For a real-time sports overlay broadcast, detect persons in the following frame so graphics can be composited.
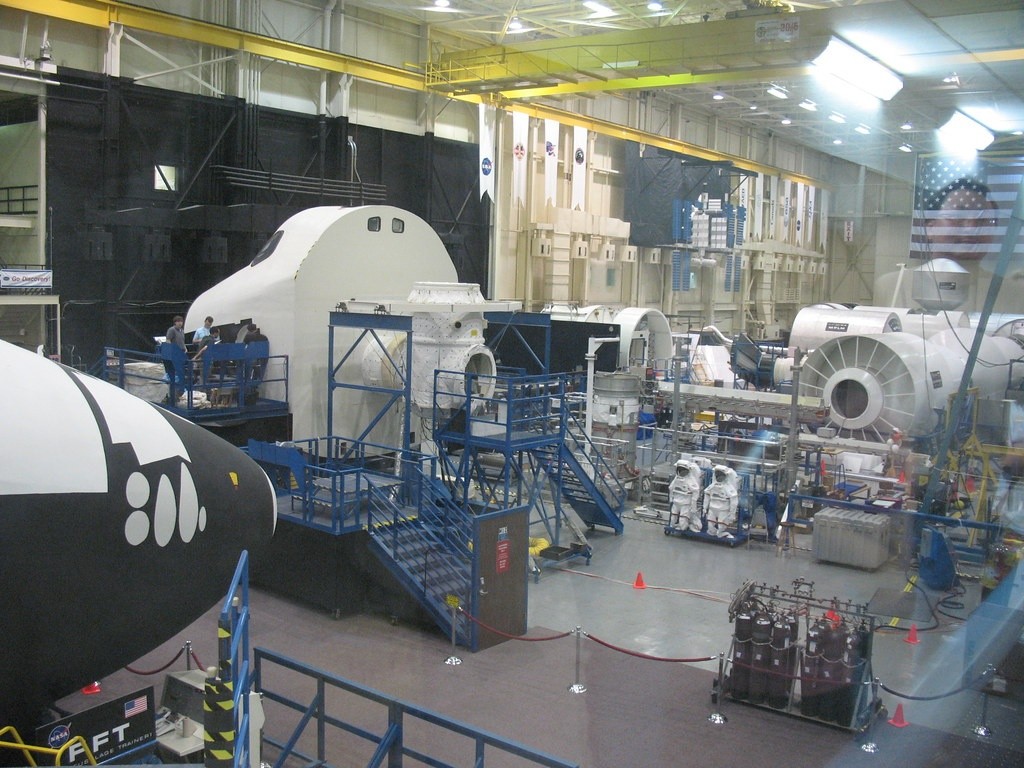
[241,323,270,395]
[701,465,739,537]
[192,315,222,379]
[668,460,701,532]
[165,315,190,402]
[876,179,1024,341]
[191,326,221,383]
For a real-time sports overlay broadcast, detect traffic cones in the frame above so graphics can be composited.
[821,459,826,475]
[903,624,920,644]
[887,702,910,729]
[897,472,905,483]
[821,609,835,620]
[967,475,975,493]
[830,615,841,631]
[633,570,648,589]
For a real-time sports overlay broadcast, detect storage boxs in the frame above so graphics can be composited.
[540,545,573,561]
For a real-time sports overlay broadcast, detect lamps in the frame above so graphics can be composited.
[767,83,789,100]
[828,111,846,124]
[898,142,912,153]
[798,98,817,112]
[855,123,871,135]
[648,0,663,11]
[509,17,522,30]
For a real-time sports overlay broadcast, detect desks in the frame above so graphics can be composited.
[155,705,205,764]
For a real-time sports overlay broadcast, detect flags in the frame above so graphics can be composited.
[909,152,1024,263]
